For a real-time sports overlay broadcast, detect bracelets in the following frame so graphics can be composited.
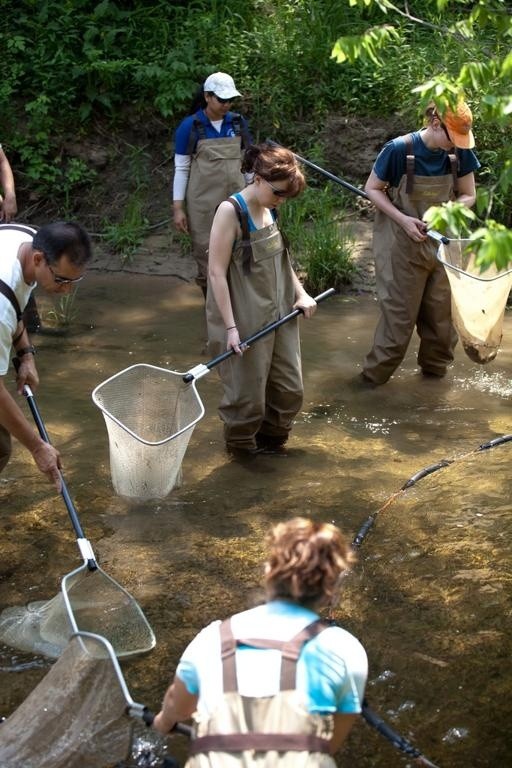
[226,325,238,329]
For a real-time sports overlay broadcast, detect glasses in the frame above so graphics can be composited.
[254,171,290,200]
[43,256,87,286]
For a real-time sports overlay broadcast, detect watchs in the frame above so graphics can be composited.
[16,344,37,358]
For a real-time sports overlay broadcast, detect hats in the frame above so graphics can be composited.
[202,71,243,99]
[434,97,476,150]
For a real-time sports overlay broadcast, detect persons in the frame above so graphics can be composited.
[0,218,94,493]
[170,69,252,295]
[203,146,320,460]
[357,99,482,386]
[1,142,19,223]
[152,518,371,768]
